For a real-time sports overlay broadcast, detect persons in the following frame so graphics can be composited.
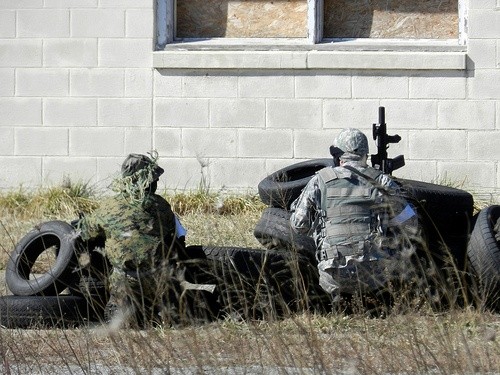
[291,127,428,316]
[107,154,224,323]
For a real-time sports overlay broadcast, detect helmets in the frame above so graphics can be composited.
[329,127,370,159]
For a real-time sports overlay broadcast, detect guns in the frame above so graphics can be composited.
[370,106,406,175]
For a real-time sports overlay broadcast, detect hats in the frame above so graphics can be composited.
[107,153,166,191]
